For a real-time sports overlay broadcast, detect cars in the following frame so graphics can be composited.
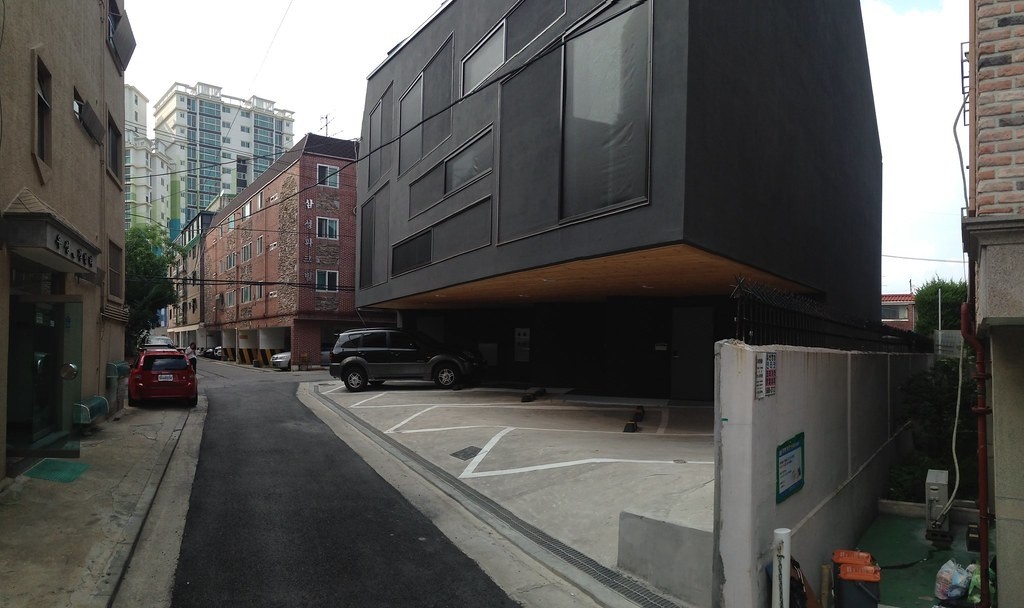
[129,348,199,406]
[269,340,332,370]
[204,345,222,358]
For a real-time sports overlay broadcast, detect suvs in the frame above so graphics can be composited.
[138,336,174,351]
[329,327,479,392]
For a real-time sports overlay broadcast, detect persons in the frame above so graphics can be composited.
[185,342,196,375]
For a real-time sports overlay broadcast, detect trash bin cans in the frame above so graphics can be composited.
[831,550,873,594]
[831,565,881,608]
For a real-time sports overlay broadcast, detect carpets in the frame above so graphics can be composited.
[24,458,91,483]
